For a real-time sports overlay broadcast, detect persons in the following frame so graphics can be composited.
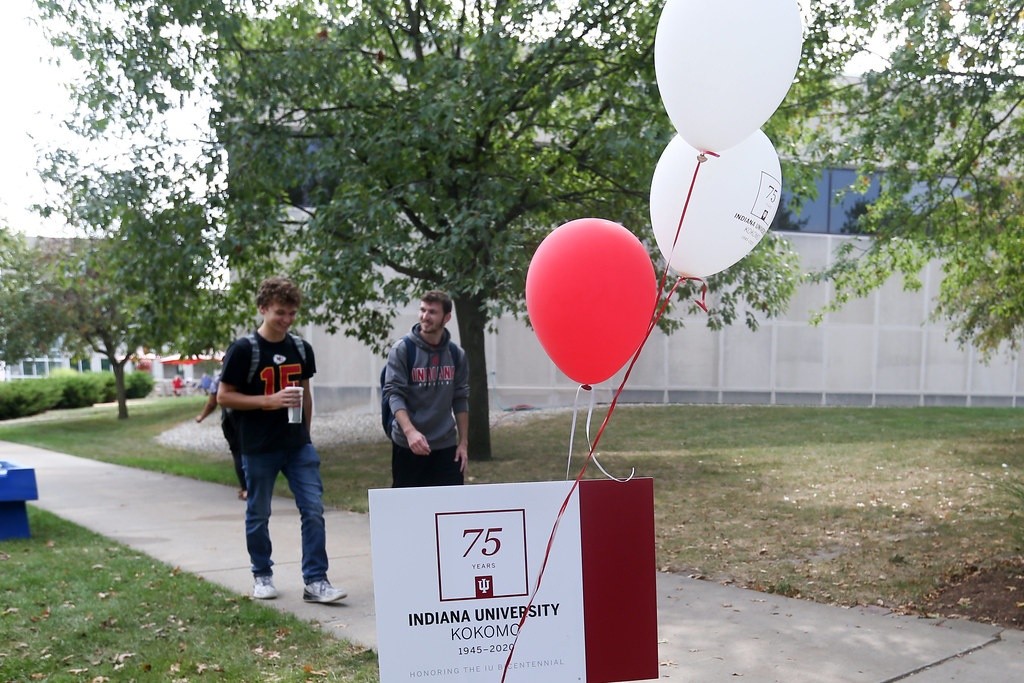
[216,278,348,603]
[196,355,248,500]
[199,371,212,396]
[381,291,471,487]
[172,377,182,396]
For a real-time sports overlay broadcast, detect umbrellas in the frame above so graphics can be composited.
[163,349,225,364]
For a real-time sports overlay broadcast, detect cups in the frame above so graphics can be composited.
[285,386,304,423]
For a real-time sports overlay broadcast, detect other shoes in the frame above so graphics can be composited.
[239,490,247,499]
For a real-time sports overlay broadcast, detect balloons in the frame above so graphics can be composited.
[525,218,658,388]
[650,127,782,277]
[654,0,803,155]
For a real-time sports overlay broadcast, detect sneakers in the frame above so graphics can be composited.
[252,575,277,599]
[303,581,347,603]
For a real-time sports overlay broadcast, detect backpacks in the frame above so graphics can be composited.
[379,335,458,439]
